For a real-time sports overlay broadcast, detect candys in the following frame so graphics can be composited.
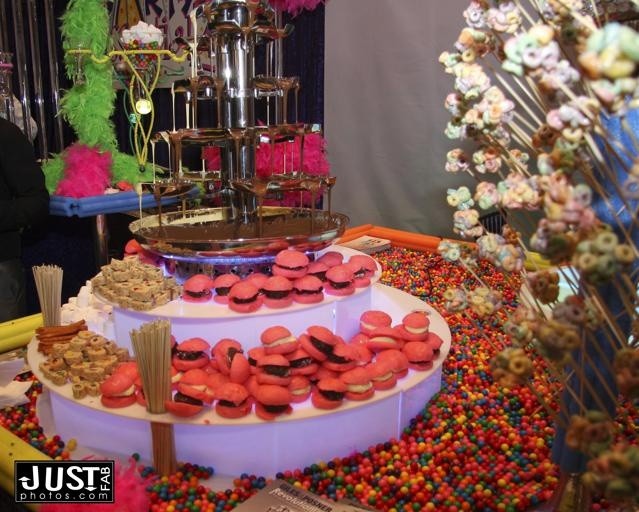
[0,0,639,512]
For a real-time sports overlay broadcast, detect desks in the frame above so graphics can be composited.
[48,180,201,271]
[0,223,573,512]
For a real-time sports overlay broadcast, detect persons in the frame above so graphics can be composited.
[0,91,37,141]
[0,117,50,322]
